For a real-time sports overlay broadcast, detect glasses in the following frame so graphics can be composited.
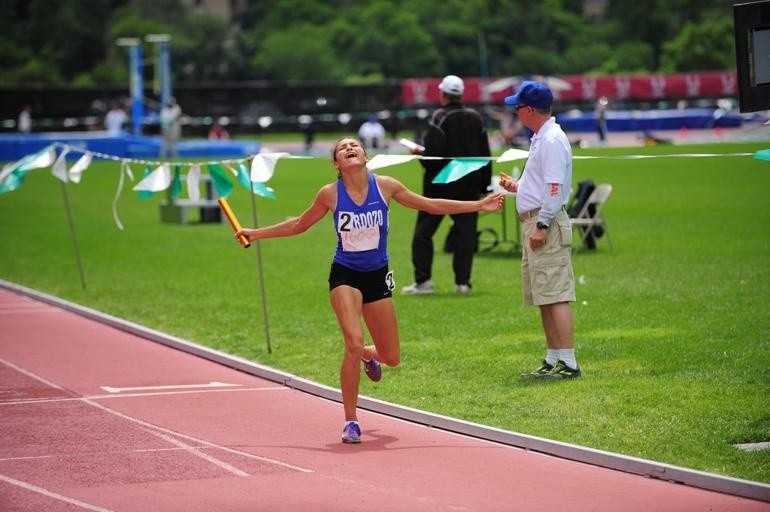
[513,104,527,111]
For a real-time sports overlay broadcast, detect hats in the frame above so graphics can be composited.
[438,75,465,96]
[505,80,553,108]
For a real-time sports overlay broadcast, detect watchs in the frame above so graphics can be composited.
[536,221,548,229]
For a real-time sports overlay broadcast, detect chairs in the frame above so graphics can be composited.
[569,176,605,247]
[570,182,614,255]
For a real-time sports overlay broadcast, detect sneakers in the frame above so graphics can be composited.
[341,422,361,444]
[359,345,382,382]
[520,359,553,379]
[456,284,470,294]
[543,359,581,378]
[400,280,434,295]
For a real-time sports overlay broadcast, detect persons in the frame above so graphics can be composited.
[16,104,34,132]
[159,96,182,163]
[103,100,129,132]
[399,74,492,296]
[498,80,581,380]
[234,135,505,444]
[207,120,230,141]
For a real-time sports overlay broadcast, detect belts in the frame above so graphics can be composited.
[519,206,541,221]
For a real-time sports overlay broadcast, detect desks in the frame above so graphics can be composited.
[494,182,524,259]
